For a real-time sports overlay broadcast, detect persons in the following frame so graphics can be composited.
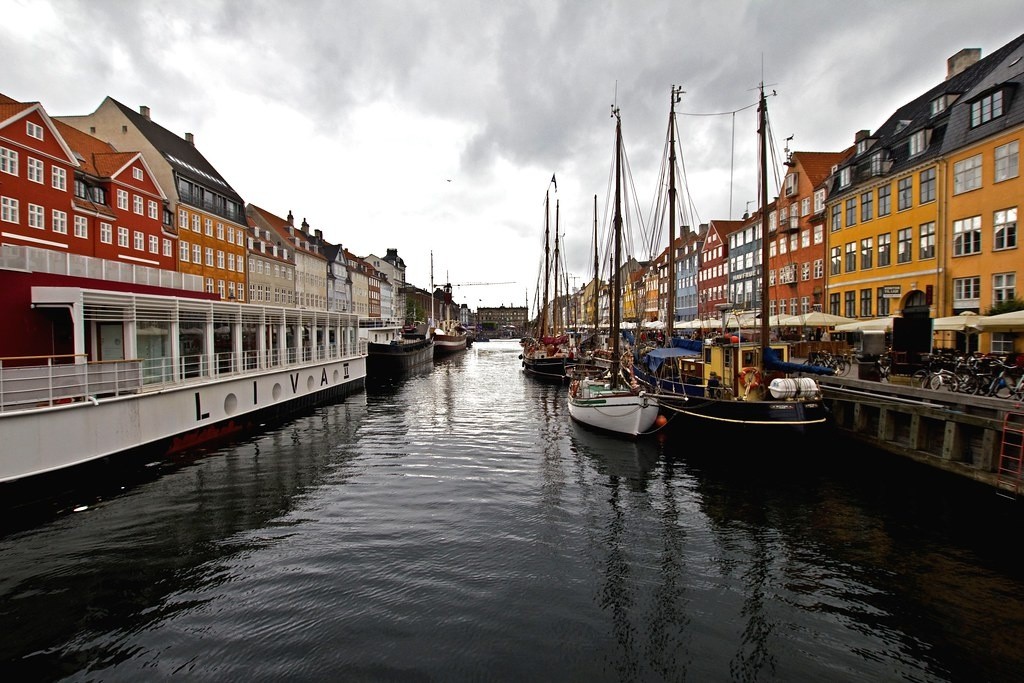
[769,328,828,343]
[622,328,734,347]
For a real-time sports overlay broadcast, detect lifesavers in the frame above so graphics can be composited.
[740,366,761,390]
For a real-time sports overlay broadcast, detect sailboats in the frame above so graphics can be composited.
[474,310,489,342]
[429,250,467,355]
[518,50,834,441]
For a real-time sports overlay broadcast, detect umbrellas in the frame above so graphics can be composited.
[563,311,858,329]
[835,314,903,333]
[933,310,988,353]
[978,310,1024,353]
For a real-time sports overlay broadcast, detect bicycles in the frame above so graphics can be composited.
[803,345,1024,402]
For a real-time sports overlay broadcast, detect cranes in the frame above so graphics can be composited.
[433,269,516,292]
